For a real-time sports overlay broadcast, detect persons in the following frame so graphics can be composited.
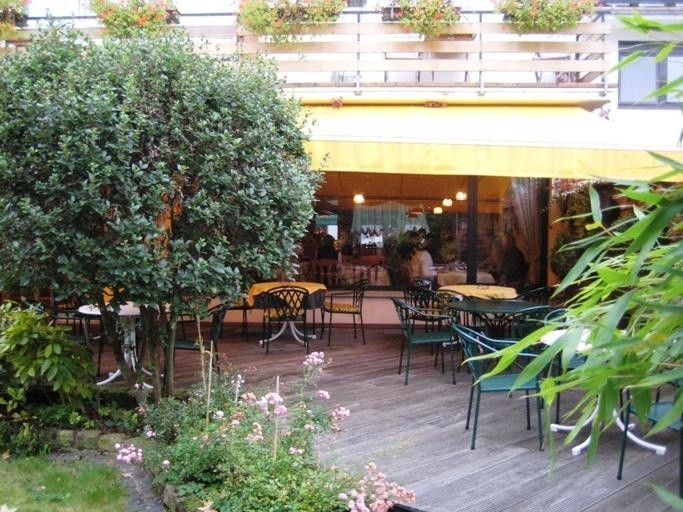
[397,228,450,288]
[477,233,528,291]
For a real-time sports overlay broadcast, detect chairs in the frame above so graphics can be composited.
[390,275,681,482]
[29,266,372,385]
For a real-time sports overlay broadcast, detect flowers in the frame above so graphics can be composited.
[84,0,174,41]
[0,0,29,42]
[390,0,460,39]
[235,0,349,48]
[493,0,600,37]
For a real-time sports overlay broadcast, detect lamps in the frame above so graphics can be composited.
[351,192,364,204]
[403,189,467,220]
